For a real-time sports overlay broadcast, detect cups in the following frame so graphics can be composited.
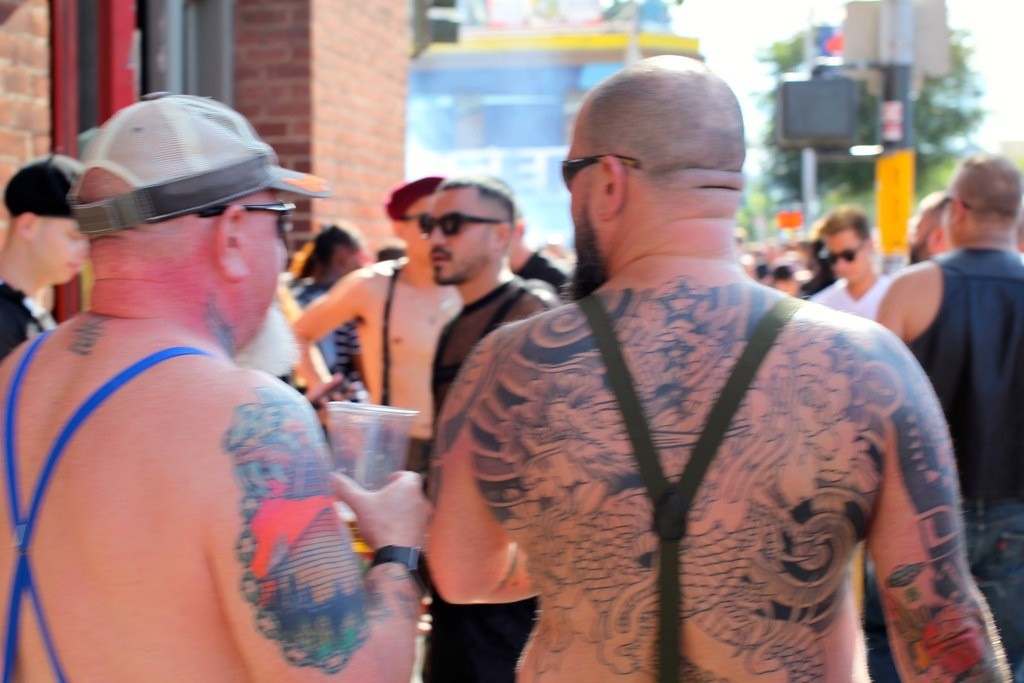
[326,400,420,552]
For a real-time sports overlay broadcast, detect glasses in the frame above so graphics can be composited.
[817,234,870,265]
[194,199,297,242]
[416,210,507,237]
[560,153,641,191]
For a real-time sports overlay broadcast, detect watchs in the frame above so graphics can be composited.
[370,544,435,598]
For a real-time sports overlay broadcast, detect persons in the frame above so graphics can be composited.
[0,90,434,683]
[428,56,1014,683]
[0,155,1024,683]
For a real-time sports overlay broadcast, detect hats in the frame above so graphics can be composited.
[5,155,87,217]
[67,91,334,233]
